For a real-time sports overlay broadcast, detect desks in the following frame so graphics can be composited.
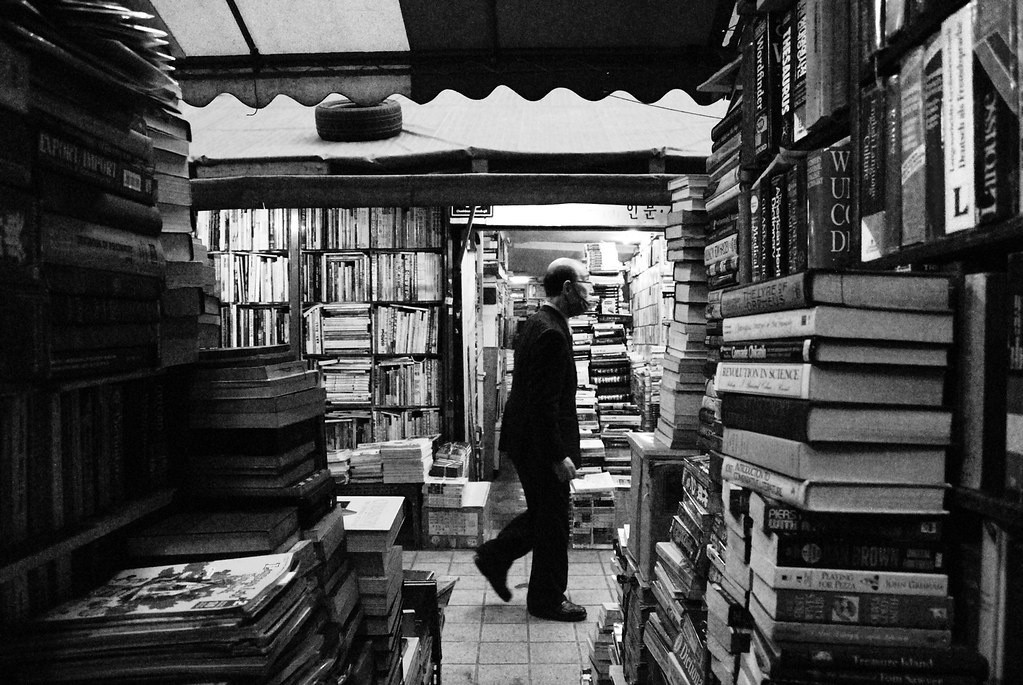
[337,482,426,551]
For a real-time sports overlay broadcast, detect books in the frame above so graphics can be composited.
[720,454,956,517]
[710,359,951,405]
[195,205,492,552]
[718,427,955,484]
[578,0,1023,685]
[483,224,674,544]
[720,270,949,314]
[719,337,951,369]
[720,305,955,347]
[0,0,456,685]
[720,393,957,449]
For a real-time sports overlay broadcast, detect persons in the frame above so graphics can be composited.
[473,257,594,621]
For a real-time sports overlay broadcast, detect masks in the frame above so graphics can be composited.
[565,286,591,317]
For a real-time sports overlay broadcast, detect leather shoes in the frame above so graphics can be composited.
[475,555,513,602]
[529,599,587,622]
